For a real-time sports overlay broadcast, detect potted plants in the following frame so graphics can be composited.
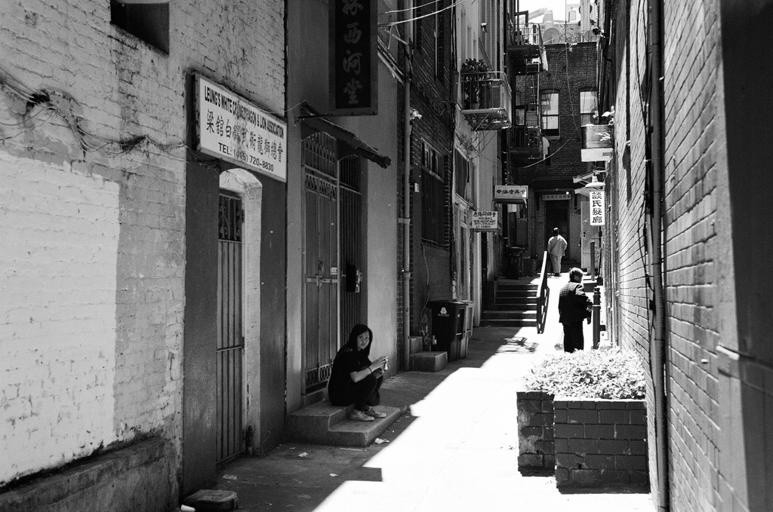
[515,345,578,478]
[549,345,650,495]
[461,57,492,110]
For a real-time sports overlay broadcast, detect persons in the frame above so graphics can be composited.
[327,324,388,422]
[558,267,594,354]
[547,227,567,276]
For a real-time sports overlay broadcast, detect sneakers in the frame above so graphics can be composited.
[347,410,375,422]
[361,406,386,419]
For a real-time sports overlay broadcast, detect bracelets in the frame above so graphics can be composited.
[368,367,373,374]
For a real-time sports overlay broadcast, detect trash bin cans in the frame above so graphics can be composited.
[426,300,474,360]
